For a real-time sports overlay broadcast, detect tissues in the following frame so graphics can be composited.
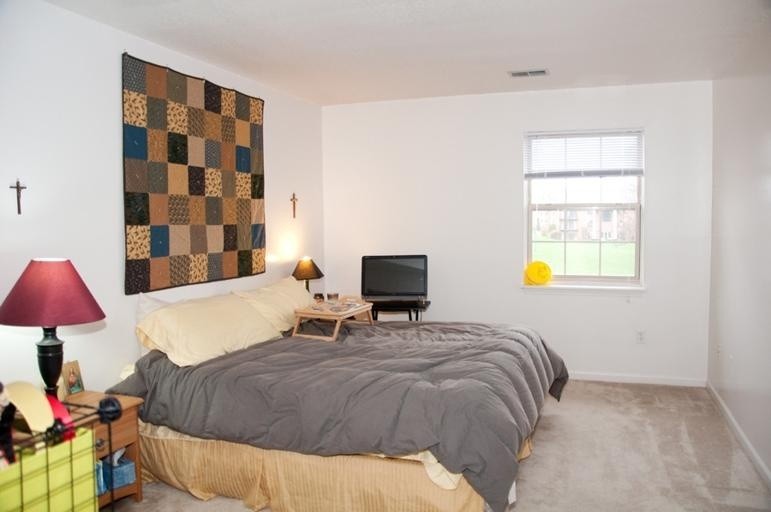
[104,448,136,490]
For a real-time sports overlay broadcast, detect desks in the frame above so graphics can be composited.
[371,301,431,319]
[294,298,373,342]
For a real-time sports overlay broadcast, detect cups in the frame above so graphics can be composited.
[327,292,338,301]
[313,293,325,304]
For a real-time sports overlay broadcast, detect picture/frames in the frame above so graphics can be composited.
[61,360,83,394]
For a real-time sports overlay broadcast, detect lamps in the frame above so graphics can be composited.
[1,258,106,402]
[293,258,324,295]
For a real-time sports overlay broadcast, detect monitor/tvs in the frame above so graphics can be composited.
[361,255,427,301]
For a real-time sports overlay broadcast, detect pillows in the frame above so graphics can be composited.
[134,277,320,369]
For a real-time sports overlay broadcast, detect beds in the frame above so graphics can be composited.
[106,320,554,511]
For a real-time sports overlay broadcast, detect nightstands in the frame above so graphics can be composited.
[61,391,145,510]
